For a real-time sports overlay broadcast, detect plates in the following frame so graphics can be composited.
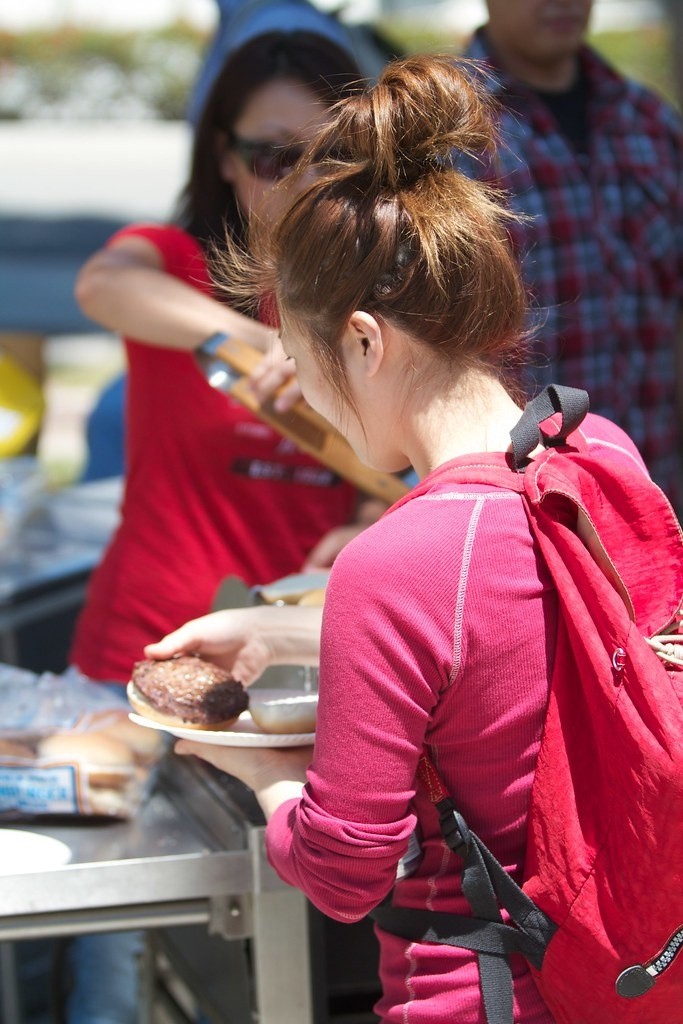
[0,829,73,876]
[261,566,333,605]
[129,688,316,748]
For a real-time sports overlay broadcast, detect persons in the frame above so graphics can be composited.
[187,1,389,129]
[60,29,367,1024]
[431,0,683,518]
[143,54,683,1024]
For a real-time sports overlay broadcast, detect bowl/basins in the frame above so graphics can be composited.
[245,687,320,732]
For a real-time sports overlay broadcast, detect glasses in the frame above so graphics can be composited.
[219,125,358,182]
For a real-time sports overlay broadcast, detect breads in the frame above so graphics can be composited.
[0,709,167,818]
[126,679,237,730]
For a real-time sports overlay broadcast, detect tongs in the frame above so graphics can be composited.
[194,332,409,503]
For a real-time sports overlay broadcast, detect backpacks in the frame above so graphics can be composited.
[368,385,683,1024]
